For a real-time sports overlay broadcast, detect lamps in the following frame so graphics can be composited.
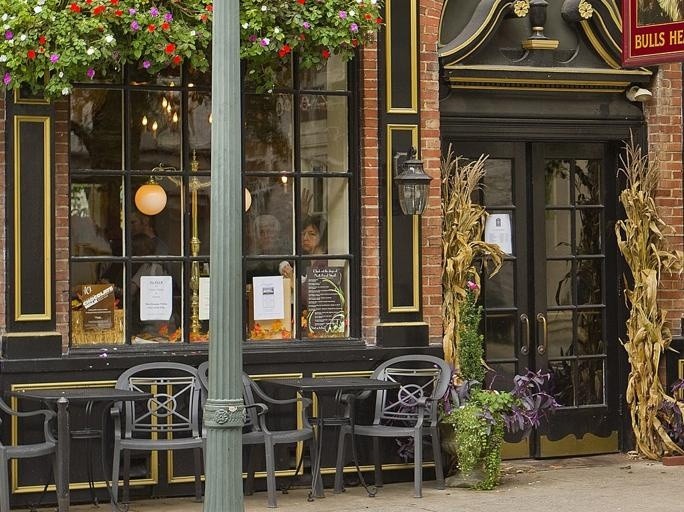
[391,145,434,217]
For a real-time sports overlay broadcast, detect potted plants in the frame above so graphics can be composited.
[438,368,562,491]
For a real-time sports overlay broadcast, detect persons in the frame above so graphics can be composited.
[94,204,183,346]
[275,214,327,309]
